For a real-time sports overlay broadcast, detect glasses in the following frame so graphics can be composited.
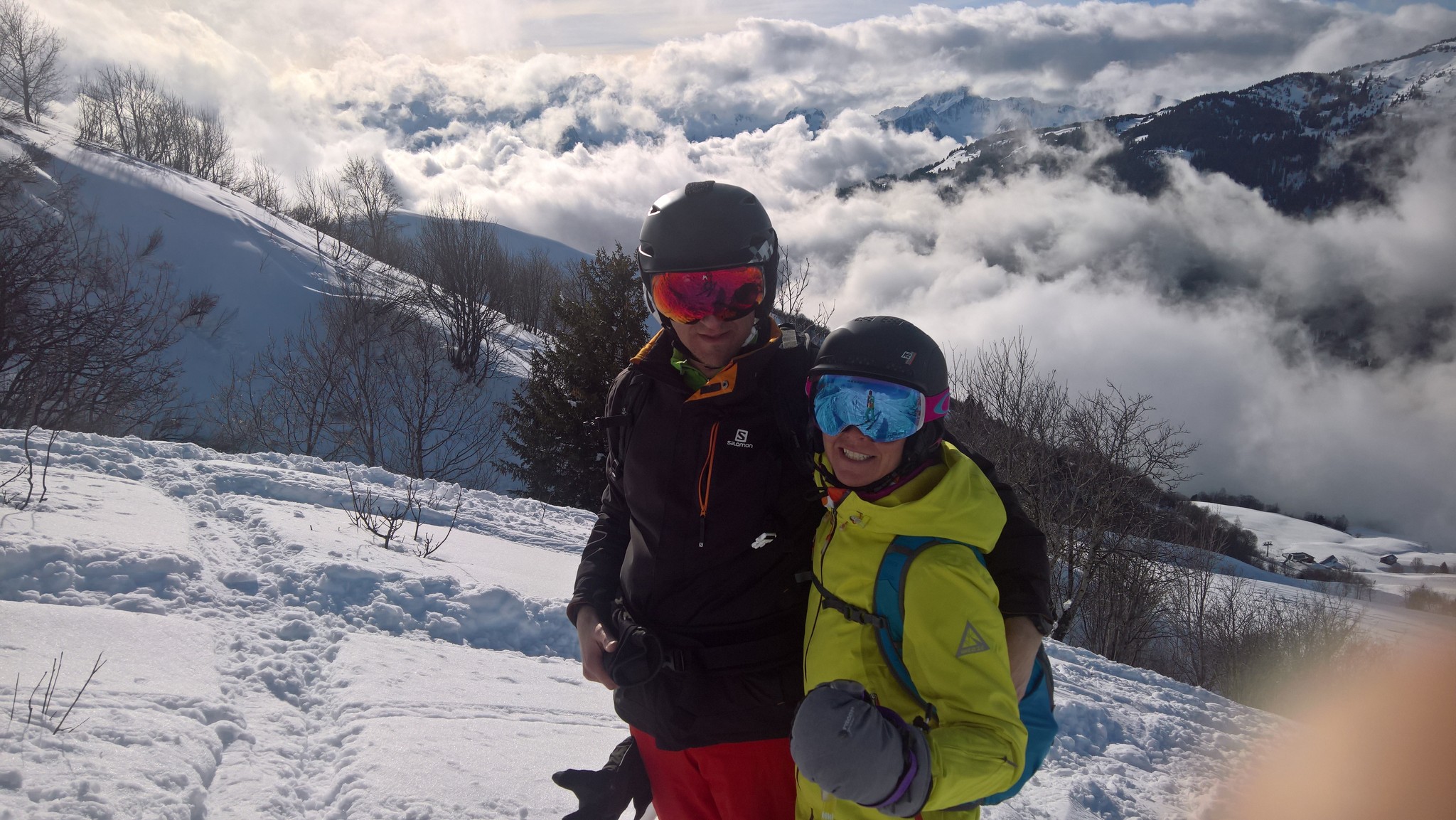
[805,371,925,444]
[647,263,767,325]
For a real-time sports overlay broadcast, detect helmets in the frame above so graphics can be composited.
[637,180,780,320]
[806,315,950,455]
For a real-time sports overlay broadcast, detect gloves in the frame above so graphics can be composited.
[790,679,933,819]
[602,582,664,688]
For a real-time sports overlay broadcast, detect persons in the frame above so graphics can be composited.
[790,316,1028,820]
[565,181,1055,820]
